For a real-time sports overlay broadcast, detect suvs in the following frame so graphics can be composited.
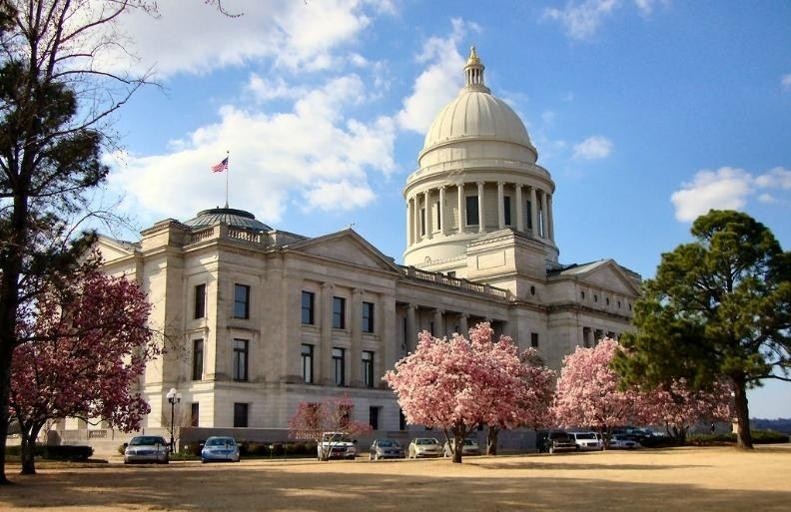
[316,432,358,460]
[538,430,578,454]
[568,431,602,450]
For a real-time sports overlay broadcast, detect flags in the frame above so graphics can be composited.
[211,156,228,172]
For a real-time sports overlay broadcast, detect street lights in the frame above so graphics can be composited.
[167,386,183,453]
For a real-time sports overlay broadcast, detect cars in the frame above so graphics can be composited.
[124,435,172,463]
[409,436,443,459]
[596,432,620,449]
[368,438,407,460]
[616,433,638,449]
[633,429,652,440]
[444,436,481,457]
[199,435,244,462]
